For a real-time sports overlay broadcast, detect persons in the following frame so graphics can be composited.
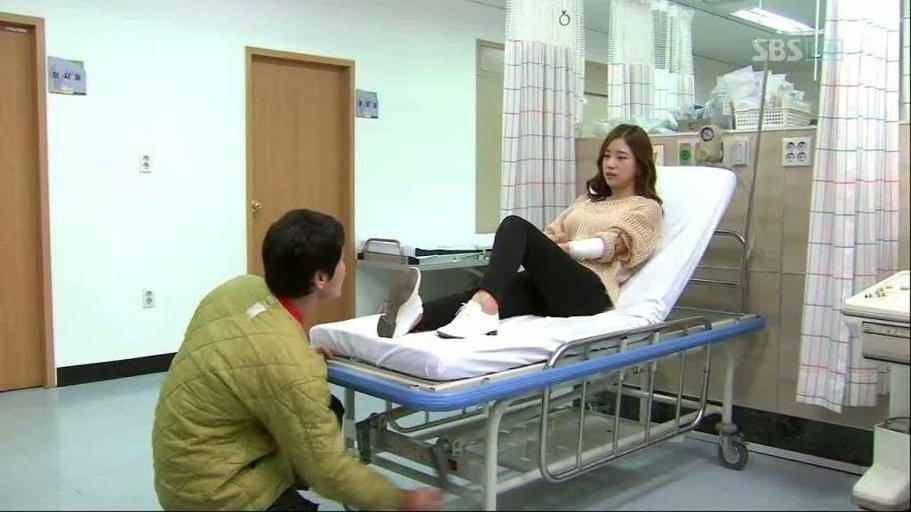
[153,208,444,511]
[376,125,666,338]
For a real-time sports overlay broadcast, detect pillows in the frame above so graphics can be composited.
[652,211,683,258]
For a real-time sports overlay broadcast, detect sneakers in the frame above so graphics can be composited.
[377,266,423,337]
[437,299,499,338]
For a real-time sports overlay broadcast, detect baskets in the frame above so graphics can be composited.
[676,115,735,132]
[733,108,812,130]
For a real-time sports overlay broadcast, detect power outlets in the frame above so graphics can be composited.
[782,137,811,167]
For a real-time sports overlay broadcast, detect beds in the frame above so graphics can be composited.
[309,166,764,511]
[358,233,496,278]
